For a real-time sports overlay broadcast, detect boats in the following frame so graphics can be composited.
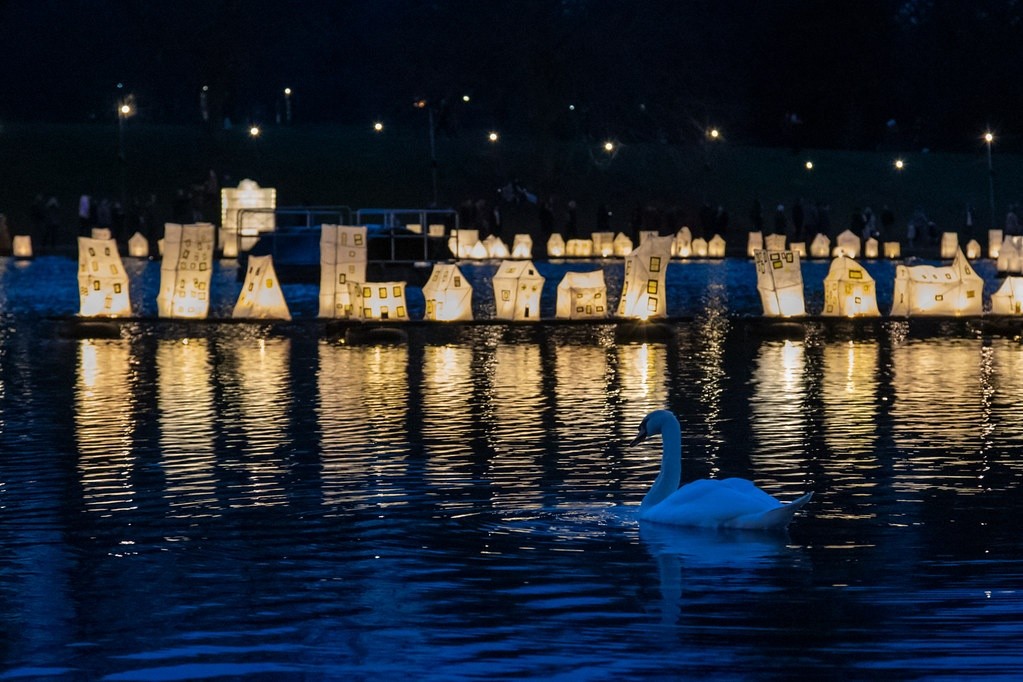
[236,204,463,287]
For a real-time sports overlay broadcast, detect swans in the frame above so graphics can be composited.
[629,409,815,532]
